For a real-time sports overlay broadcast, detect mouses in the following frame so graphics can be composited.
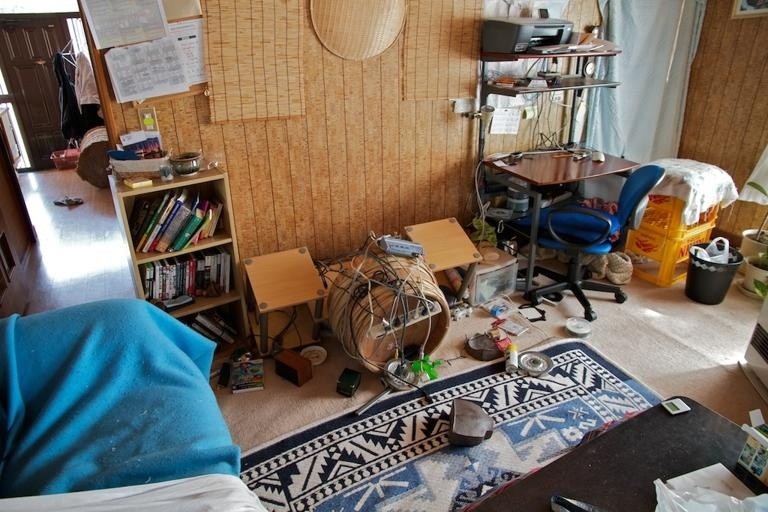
[590,151,606,163]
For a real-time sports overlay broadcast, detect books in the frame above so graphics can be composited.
[232,358,264,394]
[129,187,238,352]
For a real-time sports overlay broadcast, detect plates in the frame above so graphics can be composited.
[301,346,328,365]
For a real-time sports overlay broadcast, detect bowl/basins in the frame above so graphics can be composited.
[171,153,202,178]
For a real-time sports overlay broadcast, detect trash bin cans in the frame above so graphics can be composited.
[685,243,744,305]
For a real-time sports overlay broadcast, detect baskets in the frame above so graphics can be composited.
[50,138,81,170]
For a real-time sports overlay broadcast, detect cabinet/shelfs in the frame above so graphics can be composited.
[479,50,623,97]
[117,166,252,373]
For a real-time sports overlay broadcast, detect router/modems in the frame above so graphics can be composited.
[381,237,424,257]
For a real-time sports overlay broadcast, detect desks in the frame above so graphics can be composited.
[480,147,641,299]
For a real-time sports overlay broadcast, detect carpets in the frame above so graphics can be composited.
[236,339,664,512]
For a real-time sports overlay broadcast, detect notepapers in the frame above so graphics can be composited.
[123,177,153,189]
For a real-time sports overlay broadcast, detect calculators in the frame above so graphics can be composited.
[660,397,691,415]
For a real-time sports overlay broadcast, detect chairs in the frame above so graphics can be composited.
[513,166,666,321]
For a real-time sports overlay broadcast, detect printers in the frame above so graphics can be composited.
[482,16,575,55]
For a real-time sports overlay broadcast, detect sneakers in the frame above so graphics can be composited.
[557,249,633,284]
